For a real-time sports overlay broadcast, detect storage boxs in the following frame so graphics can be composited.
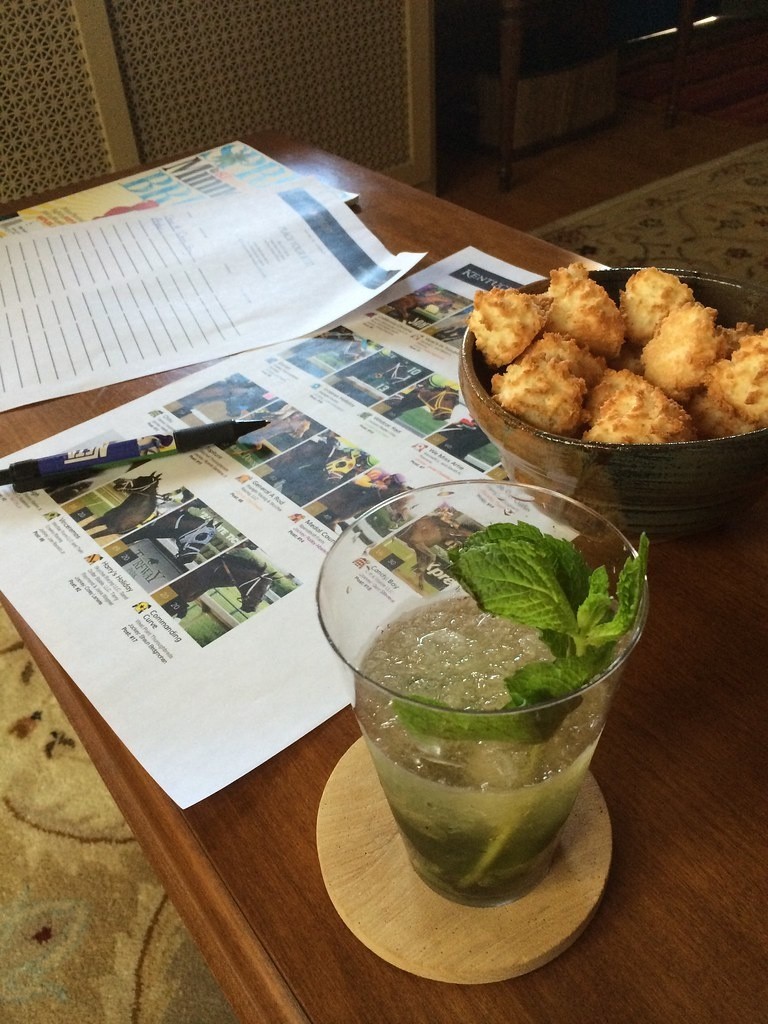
[472,48,618,162]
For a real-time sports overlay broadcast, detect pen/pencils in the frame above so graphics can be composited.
[0,419,271,494]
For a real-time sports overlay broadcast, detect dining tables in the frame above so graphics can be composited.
[0,129,768,1024]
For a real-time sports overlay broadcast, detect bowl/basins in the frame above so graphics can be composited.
[459,266,768,550]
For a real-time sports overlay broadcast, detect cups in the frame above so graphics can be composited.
[315,477,649,909]
